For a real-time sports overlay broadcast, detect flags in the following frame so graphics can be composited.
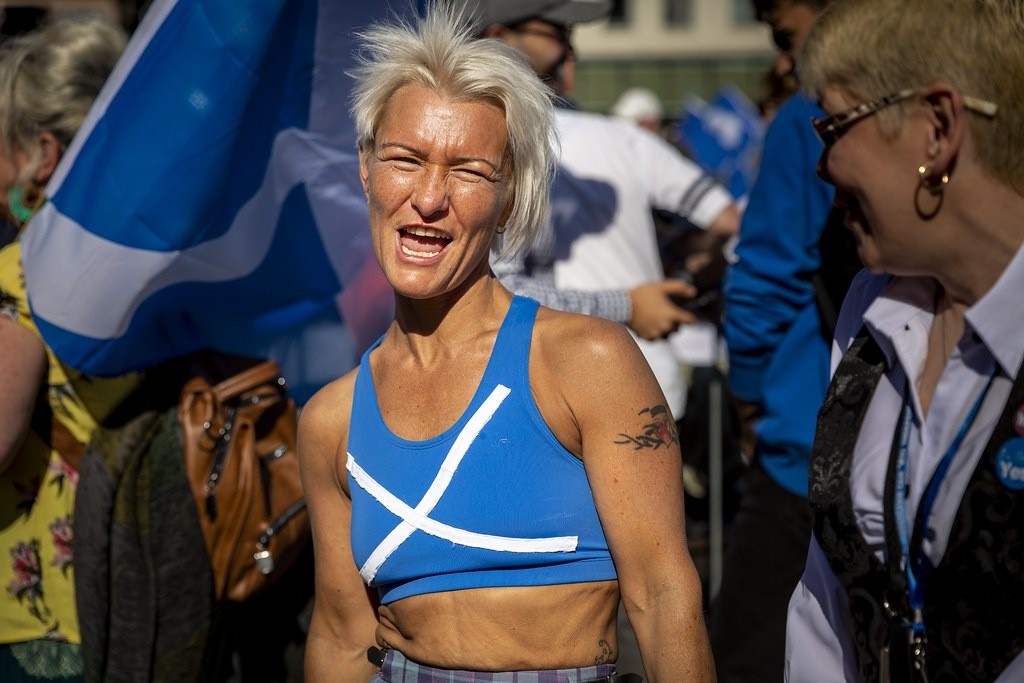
[20,0,396,405]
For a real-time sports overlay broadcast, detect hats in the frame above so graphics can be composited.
[454,1,612,38]
[611,87,667,122]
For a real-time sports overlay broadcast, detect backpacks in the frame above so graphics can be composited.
[36,345,313,682]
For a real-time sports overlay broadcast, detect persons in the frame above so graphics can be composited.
[782,0,1024,683]
[0,0,862,683]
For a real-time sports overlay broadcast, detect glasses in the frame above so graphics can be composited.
[812,84,1000,150]
